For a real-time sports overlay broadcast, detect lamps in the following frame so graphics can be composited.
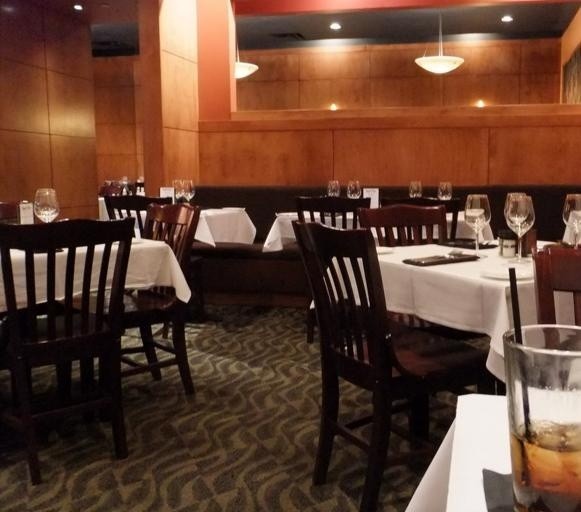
[231,2,259,79]
[414,13,464,74]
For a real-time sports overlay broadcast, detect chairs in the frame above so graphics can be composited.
[532,244,580,351]
[0,217,136,486]
[79,203,202,396]
[0,202,18,221]
[291,220,497,512]
[103,194,172,236]
[295,196,371,345]
[355,206,447,246]
[381,197,460,241]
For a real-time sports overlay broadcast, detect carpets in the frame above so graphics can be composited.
[0,291,485,512]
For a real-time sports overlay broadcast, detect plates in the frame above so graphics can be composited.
[376,247,394,254]
[482,267,534,281]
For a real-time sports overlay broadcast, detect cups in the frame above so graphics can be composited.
[409,180,422,198]
[327,180,340,197]
[182,179,195,204]
[172,180,184,203]
[34,187,60,224]
[347,180,362,199]
[503,323,581,511]
[464,193,492,259]
[504,192,536,267]
[438,181,452,200]
[564,193,581,250]
